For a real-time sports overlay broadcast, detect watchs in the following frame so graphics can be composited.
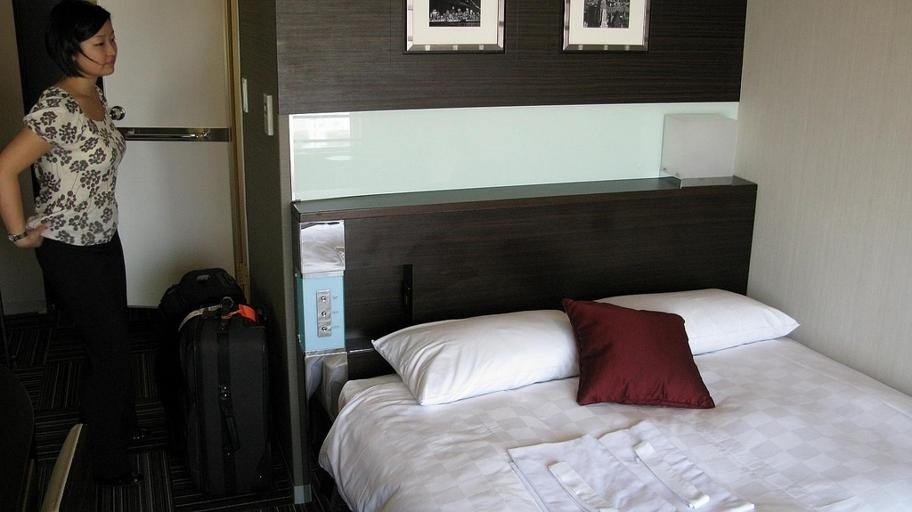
[8,232,28,242]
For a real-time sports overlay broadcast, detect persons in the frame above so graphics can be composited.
[1,2,136,491]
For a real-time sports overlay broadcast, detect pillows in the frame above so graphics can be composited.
[563,296,715,406]
[592,287,801,356]
[370,306,579,406]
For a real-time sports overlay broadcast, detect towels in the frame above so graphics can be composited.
[598,420,755,512]
[508,433,676,512]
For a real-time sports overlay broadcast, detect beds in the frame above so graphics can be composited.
[287,171,911,512]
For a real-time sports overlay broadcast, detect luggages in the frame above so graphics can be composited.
[156,267,247,417]
[179,296,282,495]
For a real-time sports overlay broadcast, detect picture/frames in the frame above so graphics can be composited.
[564,1,652,54]
[401,0,508,57]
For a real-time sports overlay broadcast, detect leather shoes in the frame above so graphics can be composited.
[129,426,152,443]
[95,472,142,487]
